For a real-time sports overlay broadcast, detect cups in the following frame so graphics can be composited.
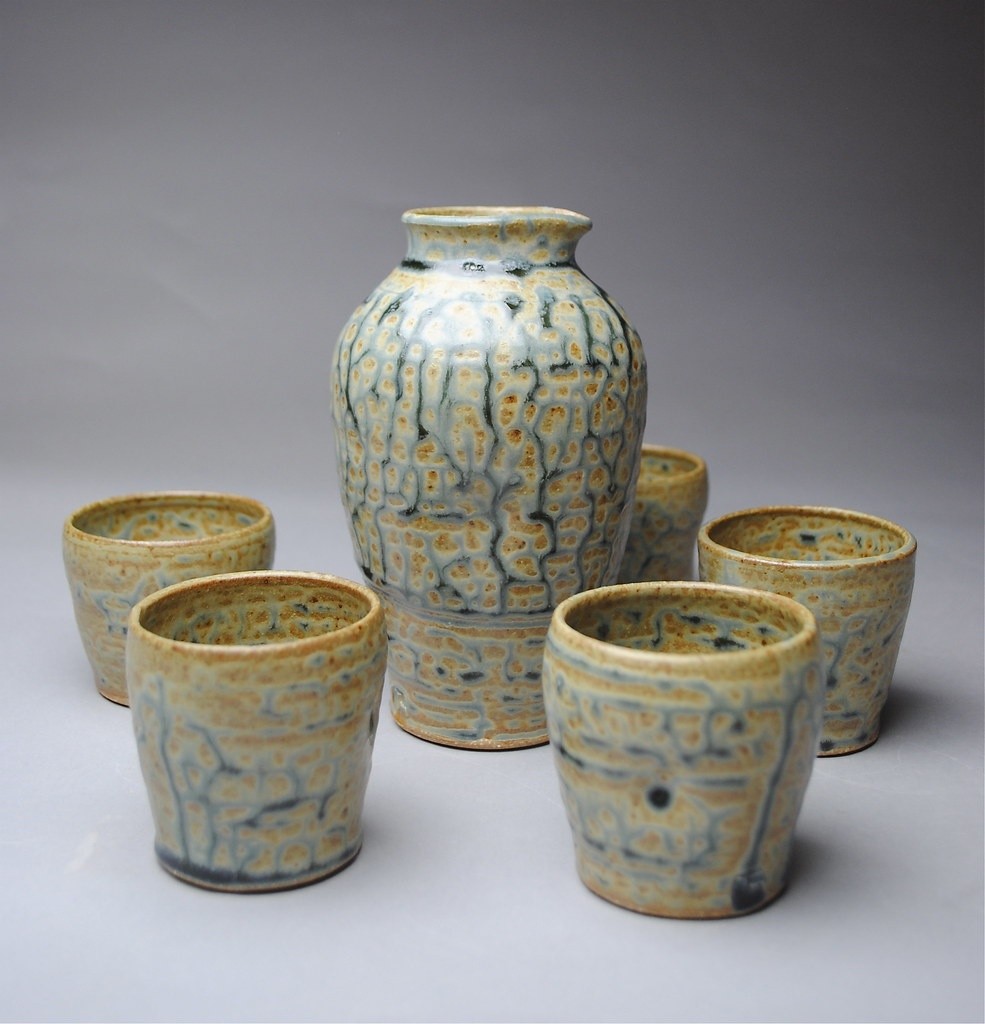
[539,584,825,918]
[693,508,917,758]
[622,444,710,583]
[124,571,387,895]
[60,491,279,711]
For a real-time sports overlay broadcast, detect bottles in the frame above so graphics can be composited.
[334,204,649,754]
